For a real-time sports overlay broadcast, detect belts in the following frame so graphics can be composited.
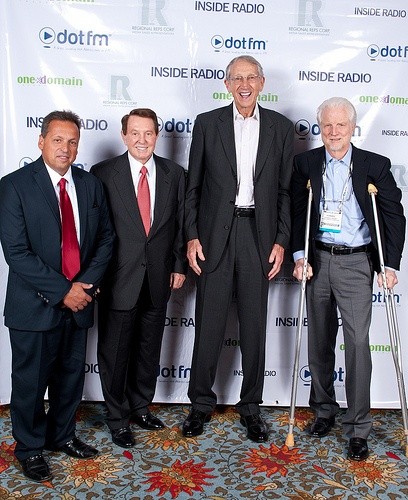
[234,207,255,217]
[314,240,371,255]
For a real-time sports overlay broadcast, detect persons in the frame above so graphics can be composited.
[293,97,407,460]
[176,55,294,440]
[0,111,98,481]
[88,108,187,446]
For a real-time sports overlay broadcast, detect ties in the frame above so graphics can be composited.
[136,165,151,238]
[56,178,80,281]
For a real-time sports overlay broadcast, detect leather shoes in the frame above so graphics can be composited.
[110,426,135,449]
[240,414,268,443]
[13,446,51,482]
[347,437,369,461]
[182,409,212,437]
[308,416,335,437]
[44,438,98,458]
[130,411,166,430]
